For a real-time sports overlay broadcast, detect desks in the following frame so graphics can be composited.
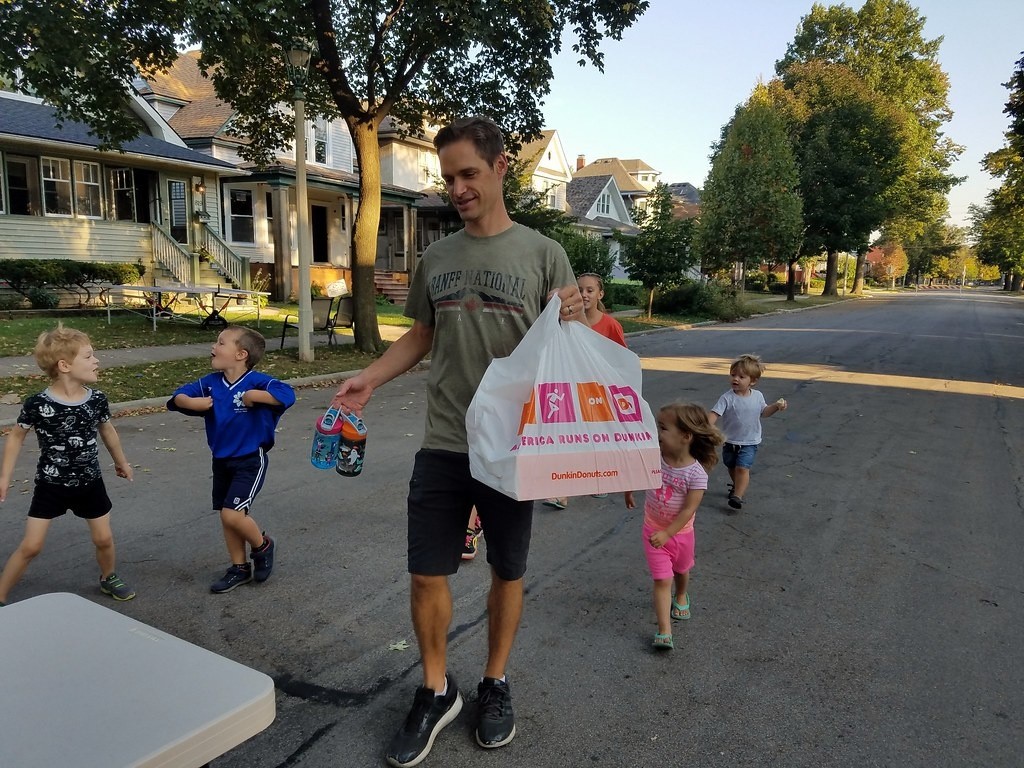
[94,284,272,331]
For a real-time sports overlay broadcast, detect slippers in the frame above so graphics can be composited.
[653,631,673,648]
[672,592,691,620]
[543,498,566,509]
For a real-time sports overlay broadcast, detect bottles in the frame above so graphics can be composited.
[336,410,367,477]
[311,403,343,469]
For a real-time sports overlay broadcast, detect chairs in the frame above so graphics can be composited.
[328,297,356,347]
[280,296,339,350]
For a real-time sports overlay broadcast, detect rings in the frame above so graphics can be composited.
[568,306,572,315]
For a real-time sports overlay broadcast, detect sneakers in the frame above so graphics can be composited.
[386,672,463,768]
[728,496,743,511]
[249,531,274,584]
[475,677,516,747]
[475,516,484,538]
[99,574,136,600]
[210,562,252,593]
[727,484,735,498]
[460,528,477,559]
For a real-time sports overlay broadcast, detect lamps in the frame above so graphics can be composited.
[195,181,207,194]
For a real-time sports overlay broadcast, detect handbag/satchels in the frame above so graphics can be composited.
[466,293,661,502]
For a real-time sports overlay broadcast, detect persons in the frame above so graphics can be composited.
[625,398,725,649]
[332,117,592,768]
[0,320,136,608]
[166,323,296,594]
[461,505,483,559]
[541,272,627,509]
[708,352,787,511]
